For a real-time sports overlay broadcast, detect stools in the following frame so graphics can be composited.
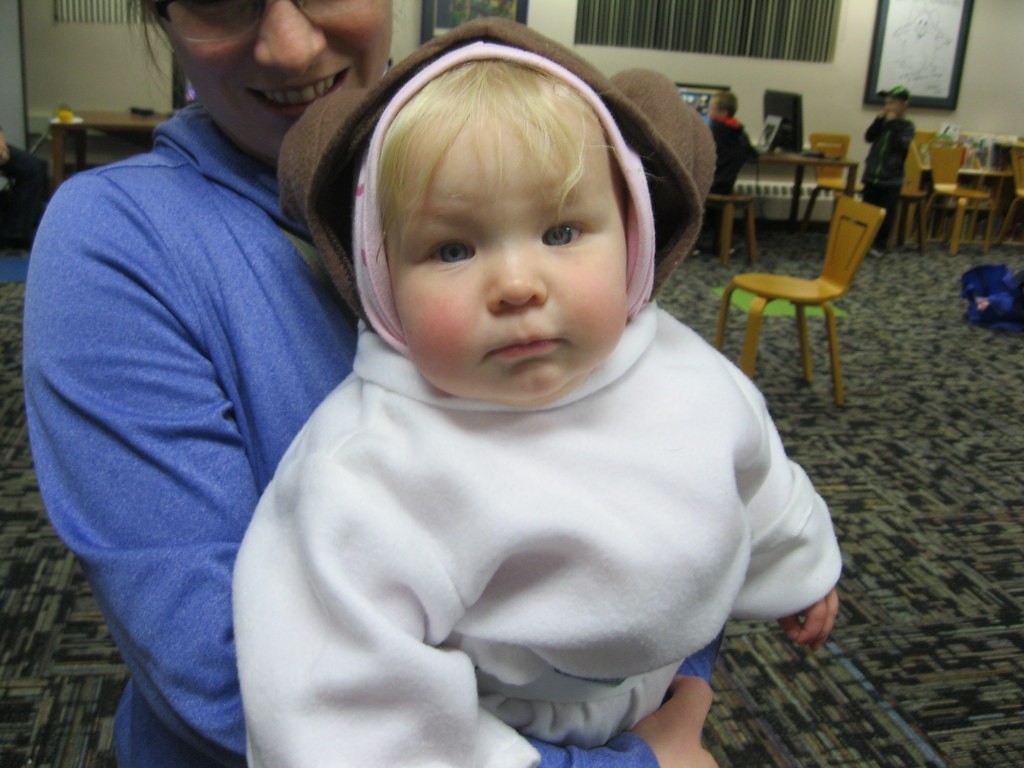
[705,194,758,267]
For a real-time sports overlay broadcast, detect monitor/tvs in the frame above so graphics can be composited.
[763,89,802,152]
[171,50,197,117]
[674,82,731,127]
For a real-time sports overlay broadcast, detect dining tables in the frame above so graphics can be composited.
[923,163,1014,245]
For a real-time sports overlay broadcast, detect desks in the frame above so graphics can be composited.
[47,106,171,192]
[763,150,860,235]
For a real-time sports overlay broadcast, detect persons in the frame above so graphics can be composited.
[688,90,757,259]
[234,12,839,765]
[24,0,722,763]
[962,263,1023,330]
[856,86,915,258]
[0,132,52,252]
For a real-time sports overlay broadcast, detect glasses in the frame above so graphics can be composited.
[148,1,378,42]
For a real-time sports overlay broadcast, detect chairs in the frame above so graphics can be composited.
[923,139,996,257]
[886,140,926,252]
[716,194,888,405]
[799,132,866,231]
[996,143,1024,247]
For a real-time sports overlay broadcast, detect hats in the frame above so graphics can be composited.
[878,86,911,107]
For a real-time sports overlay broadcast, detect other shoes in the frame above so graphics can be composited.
[713,244,736,255]
[690,244,699,256]
[868,247,885,259]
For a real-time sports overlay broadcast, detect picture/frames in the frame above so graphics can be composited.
[417,0,529,43]
[865,0,974,109]
